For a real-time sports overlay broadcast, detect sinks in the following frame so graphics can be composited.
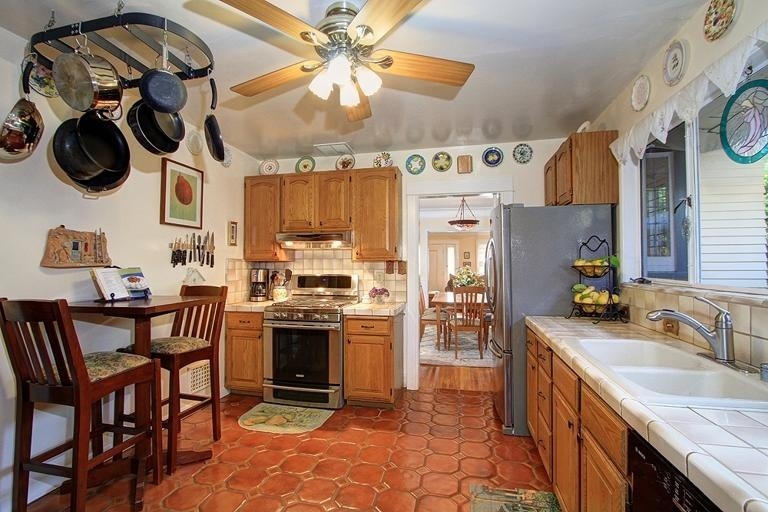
[612,367,768,409]
[560,336,716,368]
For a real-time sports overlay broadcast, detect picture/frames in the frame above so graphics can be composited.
[227,221,237,245]
[160,157,204,230]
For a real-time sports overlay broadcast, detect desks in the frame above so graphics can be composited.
[67,295,226,512]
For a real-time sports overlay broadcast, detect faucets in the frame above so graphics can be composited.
[645,296,735,362]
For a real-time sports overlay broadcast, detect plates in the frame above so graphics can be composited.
[577,121,590,133]
[702,0,735,40]
[630,75,651,113]
[661,39,687,85]
[481,143,532,168]
[257,152,452,176]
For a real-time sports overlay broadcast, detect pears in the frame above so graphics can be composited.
[175,172,192,205]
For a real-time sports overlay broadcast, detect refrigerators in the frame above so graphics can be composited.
[484,203,614,437]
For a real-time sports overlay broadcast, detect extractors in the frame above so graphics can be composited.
[277,230,354,252]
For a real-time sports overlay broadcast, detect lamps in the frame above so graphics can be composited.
[300,25,394,107]
[448,197,480,231]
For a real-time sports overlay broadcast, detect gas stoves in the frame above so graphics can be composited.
[265,295,358,323]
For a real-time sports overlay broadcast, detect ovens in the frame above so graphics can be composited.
[261,319,345,409]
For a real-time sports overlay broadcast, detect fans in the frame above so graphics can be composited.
[220,0,475,123]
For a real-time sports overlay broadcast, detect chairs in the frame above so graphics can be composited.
[420,282,492,360]
[0,298,164,512]
[113,284,228,475]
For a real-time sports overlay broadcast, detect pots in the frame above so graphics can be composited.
[1,46,227,192]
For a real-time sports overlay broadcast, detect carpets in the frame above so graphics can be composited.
[237,402,335,434]
[469,482,561,512]
[420,325,492,369]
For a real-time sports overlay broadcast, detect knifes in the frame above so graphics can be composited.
[170,230,216,268]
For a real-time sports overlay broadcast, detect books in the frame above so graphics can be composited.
[89,266,153,302]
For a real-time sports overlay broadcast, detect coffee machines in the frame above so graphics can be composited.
[249,267,269,301]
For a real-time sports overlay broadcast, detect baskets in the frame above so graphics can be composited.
[572,301,619,318]
[571,266,610,278]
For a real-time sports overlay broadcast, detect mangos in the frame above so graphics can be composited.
[575,259,608,278]
[571,284,619,313]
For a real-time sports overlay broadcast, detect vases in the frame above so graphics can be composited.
[374,295,387,306]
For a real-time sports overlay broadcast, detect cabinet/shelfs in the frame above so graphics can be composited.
[544,130,619,206]
[526,327,629,512]
[244,166,402,262]
[225,312,263,397]
[343,312,403,409]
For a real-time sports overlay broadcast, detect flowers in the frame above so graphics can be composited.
[369,286,390,298]
